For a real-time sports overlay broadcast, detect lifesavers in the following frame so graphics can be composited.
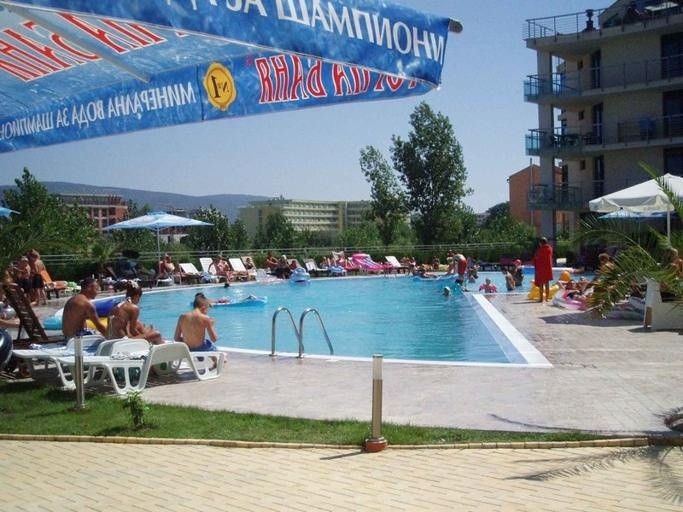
[43,317,63,330]
[291,267,309,281]
[330,265,344,274]
[83,298,120,332]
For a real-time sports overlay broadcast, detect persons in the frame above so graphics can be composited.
[670,247,683,272]
[479,277,498,293]
[245,257,255,270]
[662,247,672,267]
[401,250,480,295]
[161,253,183,272]
[174,293,219,368]
[377,260,392,265]
[62,278,107,343]
[224,283,230,288]
[528,236,553,303]
[3,247,47,307]
[264,251,297,279]
[318,255,354,269]
[109,286,164,345]
[598,252,615,271]
[208,255,231,282]
[558,280,586,301]
[502,259,524,291]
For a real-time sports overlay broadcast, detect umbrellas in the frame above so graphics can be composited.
[99,211,216,274]
[589,173,683,240]
[597,209,679,245]
